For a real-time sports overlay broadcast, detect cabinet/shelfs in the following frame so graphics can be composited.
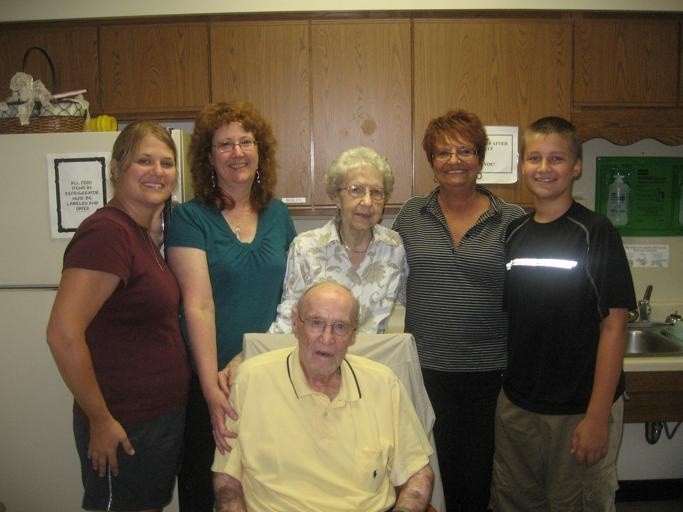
[617,372,681,496]
[3,9,681,214]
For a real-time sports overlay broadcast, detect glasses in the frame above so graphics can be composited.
[206,139,261,151]
[432,147,478,162]
[336,184,389,199]
[297,314,359,337]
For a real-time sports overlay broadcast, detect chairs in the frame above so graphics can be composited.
[240,332,447,511]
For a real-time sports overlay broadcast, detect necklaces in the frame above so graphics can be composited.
[339,223,373,253]
[231,223,241,241]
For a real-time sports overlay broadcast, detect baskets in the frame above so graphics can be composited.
[0,46,88,133]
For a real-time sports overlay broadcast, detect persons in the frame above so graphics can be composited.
[163,101,302,512]
[214,146,407,398]
[47,119,188,512]
[390,112,528,512]
[487,116,637,512]
[212,282,435,512]
